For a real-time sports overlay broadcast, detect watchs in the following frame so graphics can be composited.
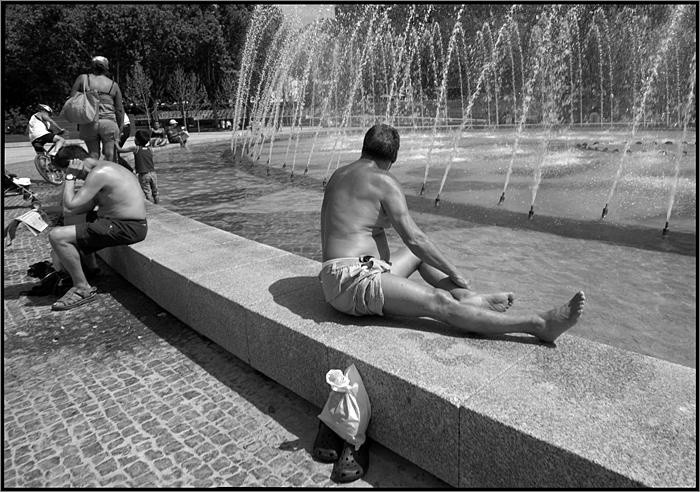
[65,173,77,181]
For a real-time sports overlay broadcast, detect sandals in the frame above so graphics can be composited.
[32,271,98,311]
[312,421,369,484]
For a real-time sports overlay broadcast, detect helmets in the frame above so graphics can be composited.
[38,103,53,117]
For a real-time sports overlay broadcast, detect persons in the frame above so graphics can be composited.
[68,56,125,163]
[113,130,159,205]
[48,144,149,311]
[148,120,167,148]
[166,119,188,148]
[77,111,138,177]
[22,103,67,157]
[321,123,585,343]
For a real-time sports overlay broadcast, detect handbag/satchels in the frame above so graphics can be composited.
[59,91,99,124]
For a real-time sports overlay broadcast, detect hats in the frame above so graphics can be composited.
[169,119,178,124]
[93,56,109,70]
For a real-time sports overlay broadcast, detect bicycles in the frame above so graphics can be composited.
[33,127,68,186]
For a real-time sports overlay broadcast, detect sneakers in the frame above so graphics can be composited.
[50,152,56,156]
